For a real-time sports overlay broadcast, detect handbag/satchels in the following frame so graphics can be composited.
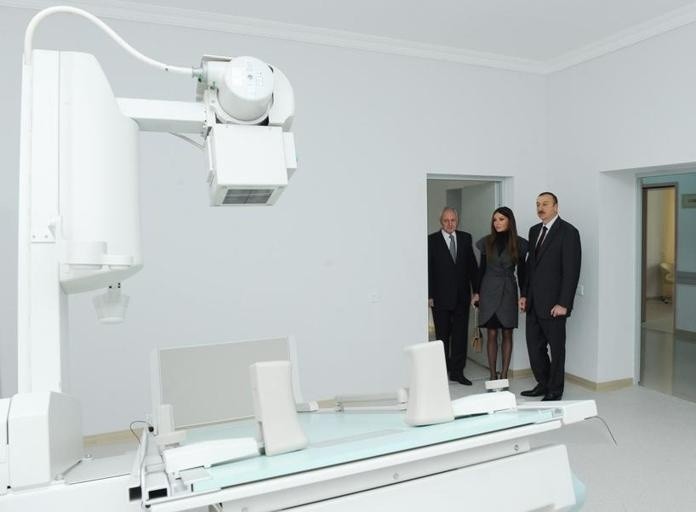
[471,306,483,353]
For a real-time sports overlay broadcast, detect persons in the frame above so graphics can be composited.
[470,206,530,391]
[519,191,582,402]
[427,204,479,386]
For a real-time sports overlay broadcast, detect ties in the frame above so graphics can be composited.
[535,226,548,258]
[449,233,456,263]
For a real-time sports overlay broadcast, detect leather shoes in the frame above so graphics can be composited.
[521,383,546,397]
[541,393,562,401]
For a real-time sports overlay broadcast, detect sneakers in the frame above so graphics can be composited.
[448,374,472,385]
